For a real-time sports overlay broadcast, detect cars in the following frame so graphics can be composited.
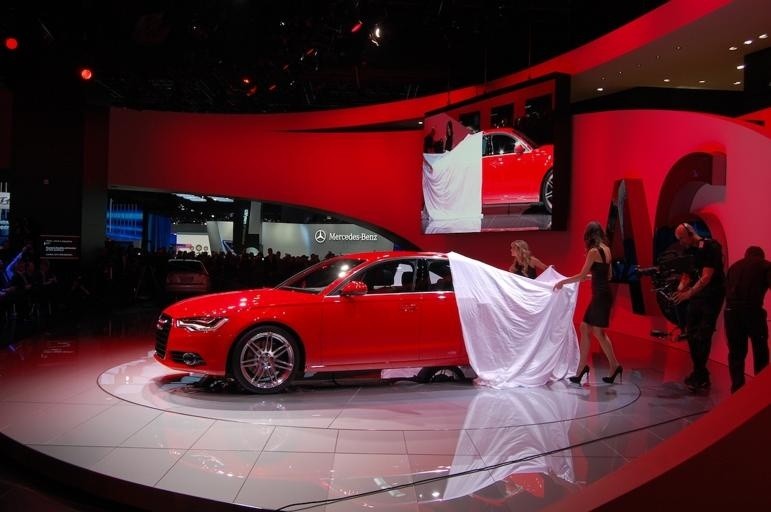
[482,128,555,215]
[164,258,209,292]
[154,251,469,394]
[155,397,469,508]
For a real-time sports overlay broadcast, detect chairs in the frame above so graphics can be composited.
[399,270,415,292]
[378,269,396,293]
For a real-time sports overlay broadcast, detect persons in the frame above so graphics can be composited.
[721,247,771,393]
[509,240,556,279]
[492,110,540,137]
[424,120,453,153]
[554,221,623,384]
[1,241,76,323]
[164,246,334,291]
[672,223,727,384]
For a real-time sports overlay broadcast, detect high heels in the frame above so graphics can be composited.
[569,364,590,383]
[602,364,623,384]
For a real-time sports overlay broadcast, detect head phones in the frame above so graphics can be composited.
[680,222,694,238]
